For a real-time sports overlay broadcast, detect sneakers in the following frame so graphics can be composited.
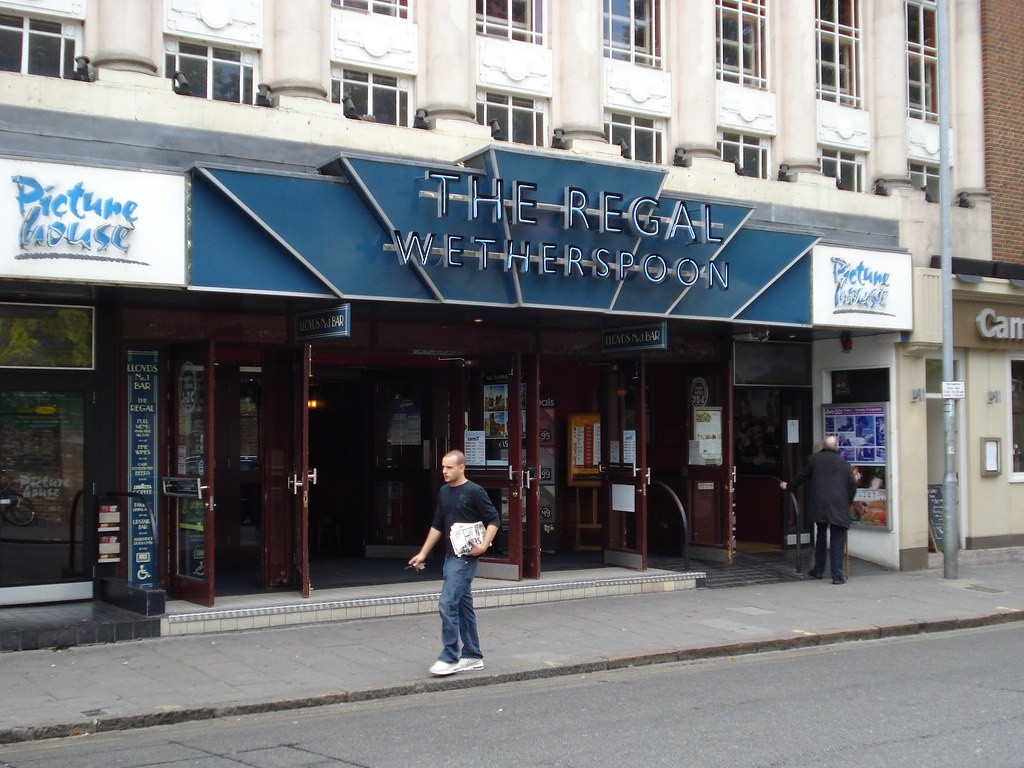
[429,661,459,676]
[458,657,484,671]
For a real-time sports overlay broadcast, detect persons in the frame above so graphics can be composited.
[856,417,874,446]
[839,434,849,446]
[838,417,853,432]
[780,435,857,584]
[407,450,502,675]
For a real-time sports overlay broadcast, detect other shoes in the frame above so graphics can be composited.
[833,579,845,584]
[810,569,822,579]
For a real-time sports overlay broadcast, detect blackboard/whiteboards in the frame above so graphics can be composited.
[928,484,943,553]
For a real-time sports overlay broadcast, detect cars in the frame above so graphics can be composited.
[184,456,263,526]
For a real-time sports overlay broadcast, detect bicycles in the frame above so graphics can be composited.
[0,482,35,527]
